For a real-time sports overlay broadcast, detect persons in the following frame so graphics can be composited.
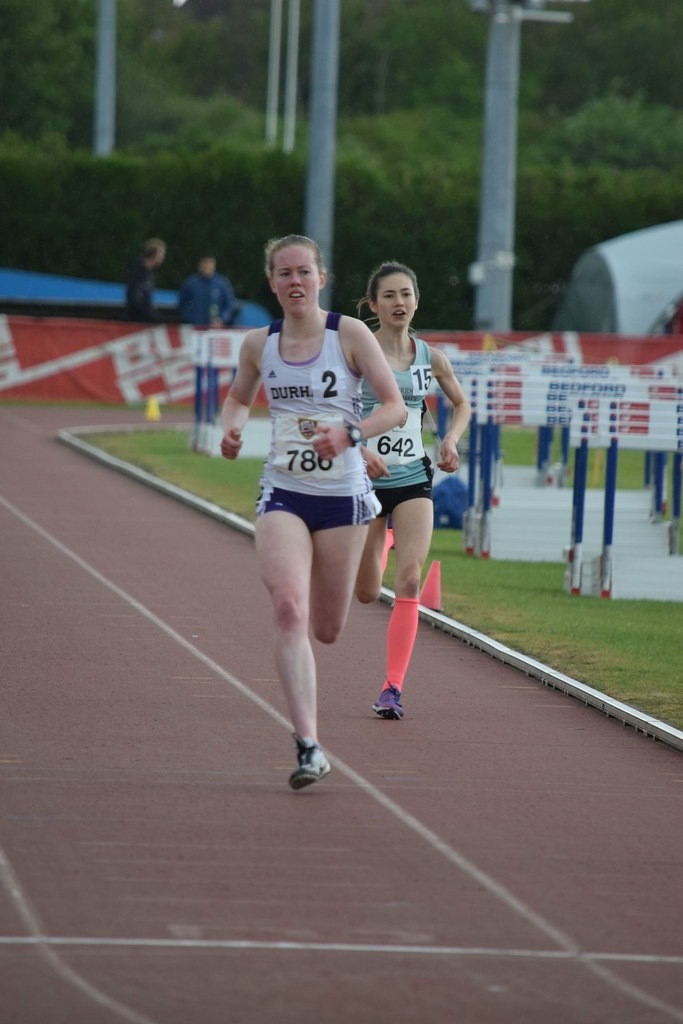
[128,239,165,319]
[221,235,407,790]
[175,255,234,327]
[355,263,472,719]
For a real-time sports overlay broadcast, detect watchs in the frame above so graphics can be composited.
[346,424,364,446]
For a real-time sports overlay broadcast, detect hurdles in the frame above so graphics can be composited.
[183,326,683,600]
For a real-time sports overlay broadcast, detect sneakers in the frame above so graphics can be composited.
[288,732,330,790]
[371,686,405,720]
